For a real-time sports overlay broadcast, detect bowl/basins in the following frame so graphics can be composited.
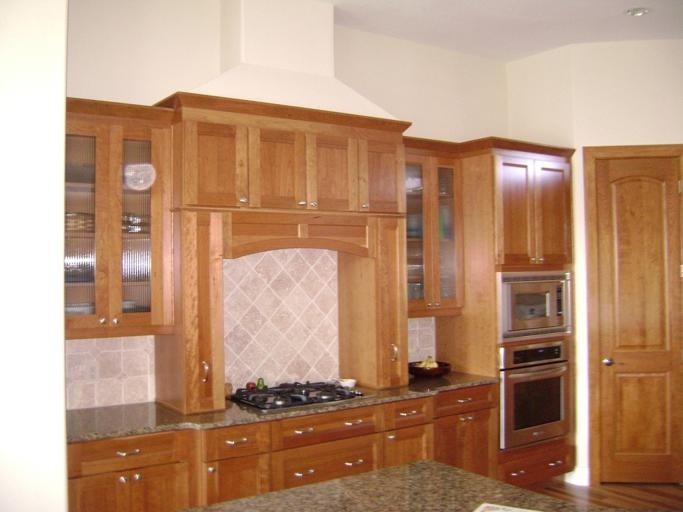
[338,378,357,387]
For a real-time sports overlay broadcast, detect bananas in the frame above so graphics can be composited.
[415,356,438,368]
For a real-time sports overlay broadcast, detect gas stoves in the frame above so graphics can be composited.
[223,382,362,412]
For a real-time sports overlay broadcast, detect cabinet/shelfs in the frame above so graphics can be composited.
[272,403,384,492]
[501,441,568,487]
[432,383,499,483]
[458,140,574,276]
[382,397,434,467]
[66,430,202,512]
[176,89,407,216]
[65,97,178,334]
[403,137,463,317]
[200,421,272,507]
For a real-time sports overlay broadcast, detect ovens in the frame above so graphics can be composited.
[497,339,571,450]
[495,269,573,344]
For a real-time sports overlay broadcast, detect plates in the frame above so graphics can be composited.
[408,361,451,379]
[124,163,156,191]
[64,211,94,232]
